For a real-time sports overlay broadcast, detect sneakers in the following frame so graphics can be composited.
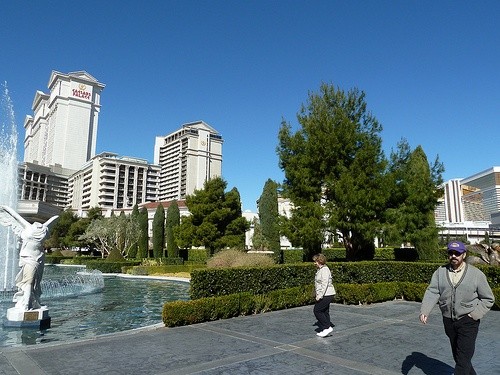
[316,326,334,338]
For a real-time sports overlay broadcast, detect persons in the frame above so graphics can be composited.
[488,242,500,266]
[310,254,337,338]
[15,221,50,310]
[419,240,496,375]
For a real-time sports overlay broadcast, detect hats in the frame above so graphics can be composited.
[447,241,467,254]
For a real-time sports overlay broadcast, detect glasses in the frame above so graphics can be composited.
[447,250,462,257]
